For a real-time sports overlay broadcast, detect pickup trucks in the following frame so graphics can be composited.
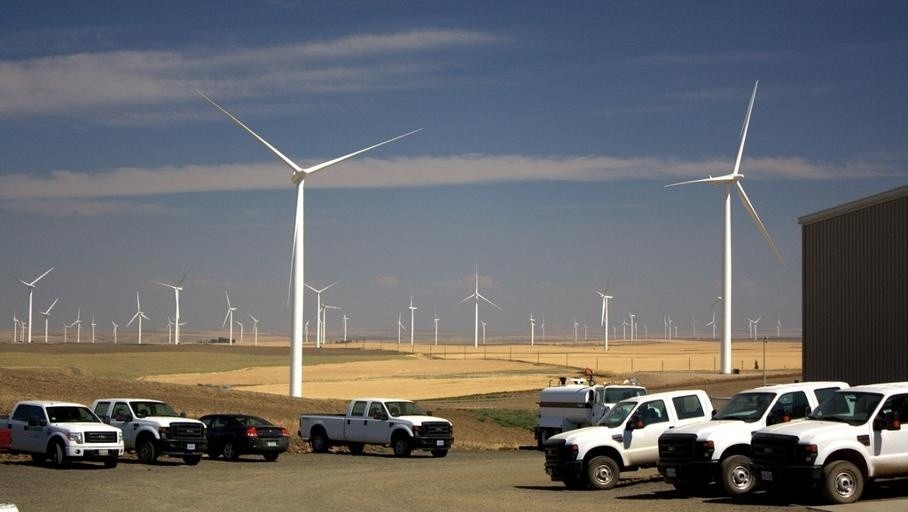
[0,399,125,468]
[298,398,454,457]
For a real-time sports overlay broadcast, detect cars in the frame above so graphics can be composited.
[199,415,290,463]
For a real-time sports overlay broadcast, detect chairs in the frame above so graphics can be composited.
[770,401,785,416]
[372,407,379,415]
[647,408,655,419]
[392,407,399,414]
[867,400,881,419]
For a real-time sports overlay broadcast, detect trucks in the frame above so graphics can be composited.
[533,382,647,451]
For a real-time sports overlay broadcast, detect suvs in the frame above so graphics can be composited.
[93,394,210,467]
[540,390,714,490]
[652,380,856,494]
[754,380,908,505]
